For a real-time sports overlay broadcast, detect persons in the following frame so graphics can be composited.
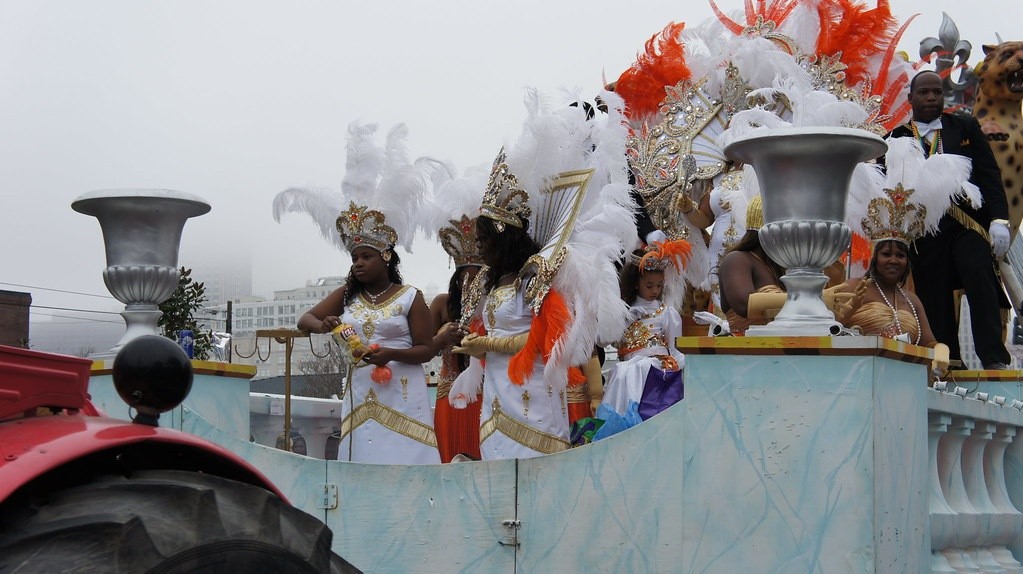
[568,65,844,424]
[876,70,1012,372]
[430,147,570,463]
[297,200,443,464]
[835,182,950,370]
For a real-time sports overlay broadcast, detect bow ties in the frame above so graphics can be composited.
[914,118,943,137]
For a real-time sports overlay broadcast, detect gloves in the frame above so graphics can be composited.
[840,276,867,323]
[989,219,1010,258]
[747,283,856,320]
[926,342,950,373]
[676,194,697,217]
[451,332,529,357]
[461,334,486,359]
[582,355,603,415]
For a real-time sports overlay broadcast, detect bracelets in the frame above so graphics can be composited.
[993,219,1011,228]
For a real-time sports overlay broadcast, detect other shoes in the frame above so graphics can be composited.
[987,363,1005,381]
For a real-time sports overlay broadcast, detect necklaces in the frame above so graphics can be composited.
[364,282,394,306]
[499,273,512,281]
[874,280,921,346]
[911,115,942,154]
